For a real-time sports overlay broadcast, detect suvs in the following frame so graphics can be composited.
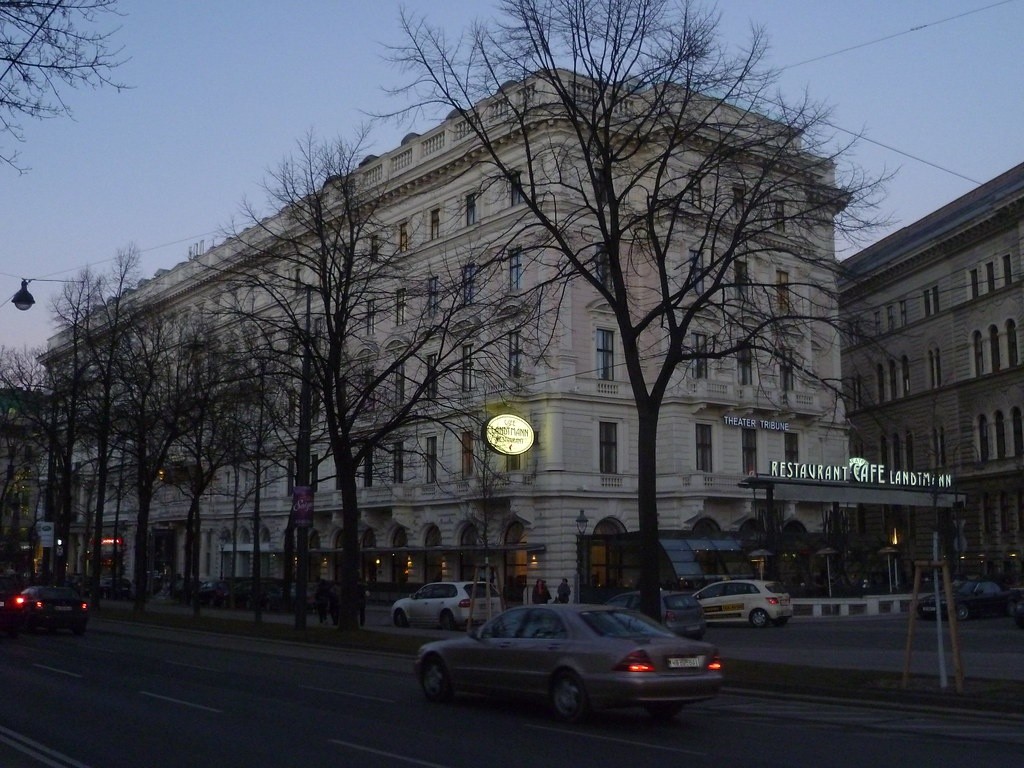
[390,581,506,632]
[0,574,26,637]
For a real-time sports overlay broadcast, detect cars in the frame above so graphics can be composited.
[195,580,230,604]
[414,603,723,723]
[100,577,132,599]
[155,579,202,604]
[224,581,280,606]
[1014,591,1024,630]
[60,573,89,591]
[601,590,706,642]
[268,582,326,612]
[23,586,90,634]
[917,580,1020,621]
[676,580,794,628]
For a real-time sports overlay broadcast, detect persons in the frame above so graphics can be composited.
[44,572,55,586]
[558,579,571,603]
[357,577,366,626]
[38,569,42,579]
[315,579,341,626]
[532,579,552,604]
